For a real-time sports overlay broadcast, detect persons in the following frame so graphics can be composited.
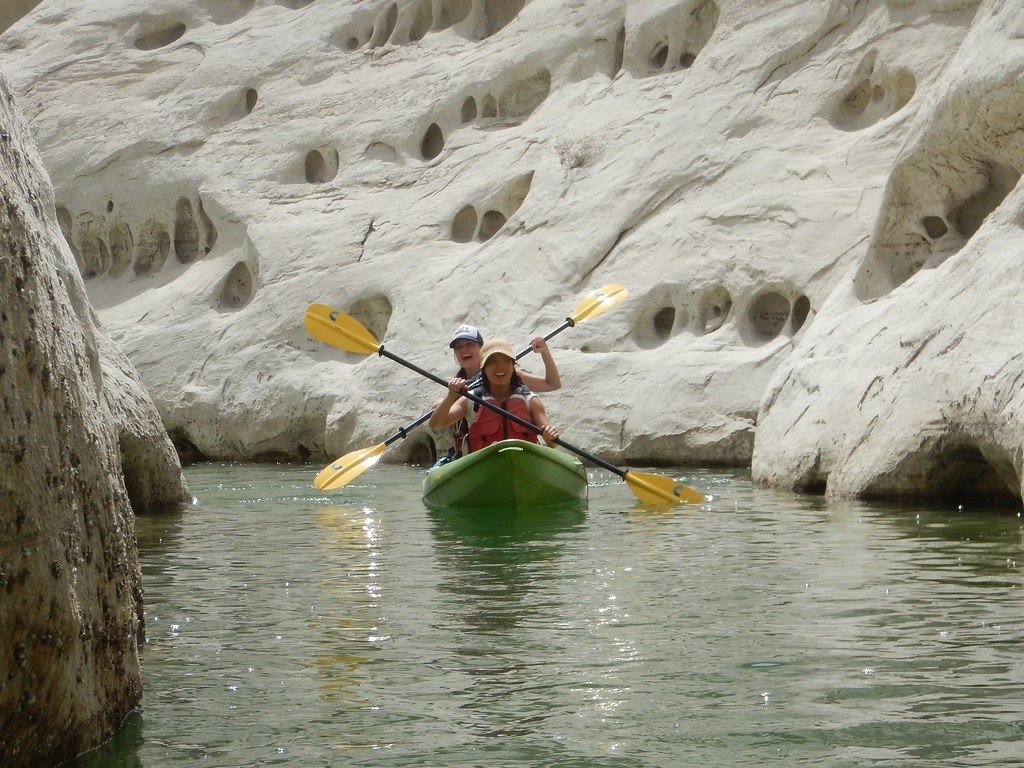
[432,324,561,458]
[429,338,559,453]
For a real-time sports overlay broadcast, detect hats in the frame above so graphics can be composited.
[449,325,484,348]
[477,338,516,368]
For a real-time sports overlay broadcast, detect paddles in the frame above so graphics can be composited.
[303,303,705,506]
[311,283,628,492]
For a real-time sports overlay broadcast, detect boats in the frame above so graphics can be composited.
[421,439,589,511]
